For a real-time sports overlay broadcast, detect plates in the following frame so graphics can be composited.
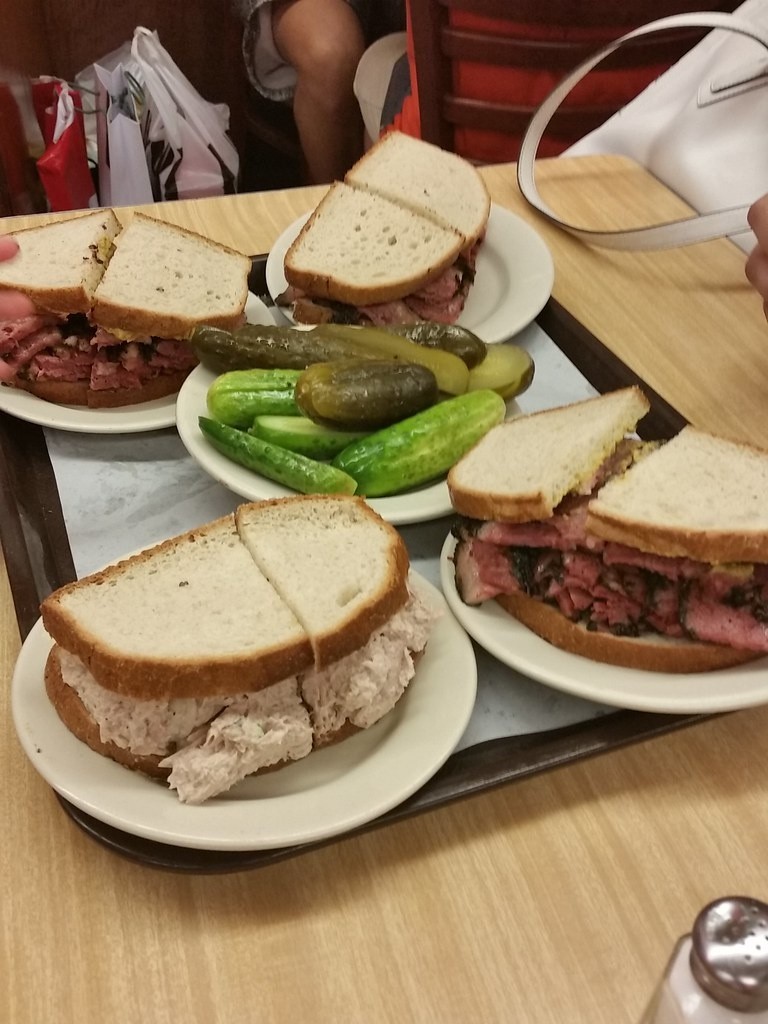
[440,527,768,715]
[0,291,276,433]
[265,202,554,345]
[175,359,524,525]
[10,569,477,852]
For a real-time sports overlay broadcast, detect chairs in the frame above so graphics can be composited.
[405,0,737,171]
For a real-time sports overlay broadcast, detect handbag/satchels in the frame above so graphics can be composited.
[74,25,240,208]
[30,74,99,213]
[516,0,768,256]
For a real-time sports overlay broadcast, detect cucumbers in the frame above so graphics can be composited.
[207,369,298,426]
[334,386,506,497]
[199,416,357,498]
[248,416,370,458]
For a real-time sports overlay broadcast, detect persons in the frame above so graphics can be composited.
[745,193,768,320]
[243,1,364,184]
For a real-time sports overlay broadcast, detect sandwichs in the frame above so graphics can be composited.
[1,210,250,408]
[283,130,490,325]
[449,388,767,672]
[40,496,444,803]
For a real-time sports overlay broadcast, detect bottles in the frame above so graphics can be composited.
[641,896,767,1023]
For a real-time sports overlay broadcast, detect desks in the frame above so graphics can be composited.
[0,150,768,1024]
